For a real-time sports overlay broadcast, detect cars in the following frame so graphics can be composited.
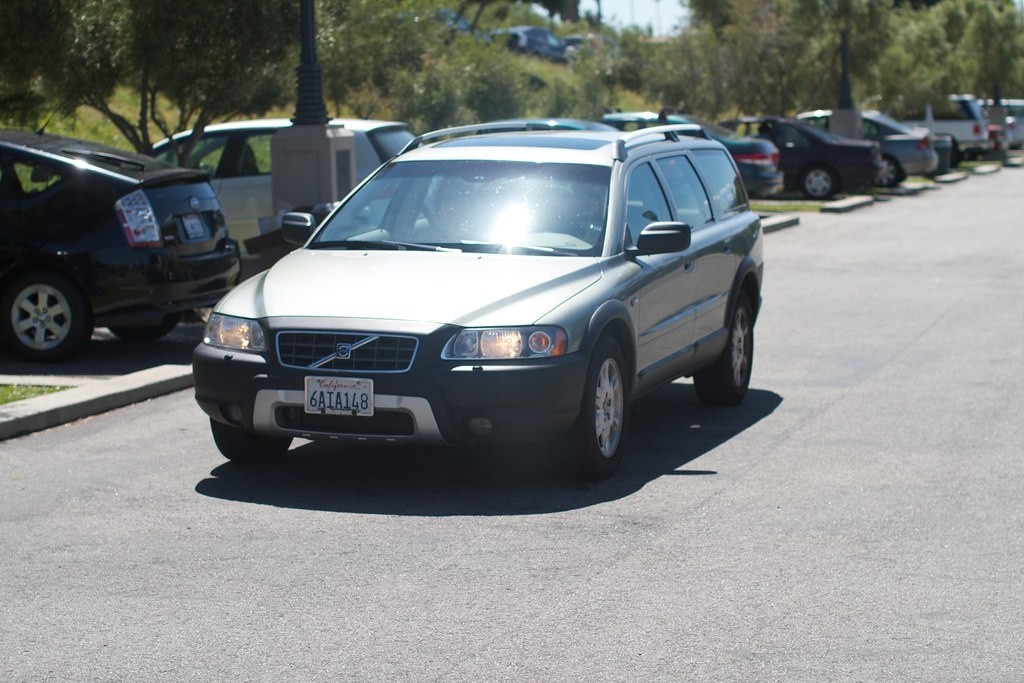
[603,113,783,197]
[718,119,883,200]
[420,8,617,68]
[0,124,239,363]
[803,112,939,190]
[140,118,419,276]
[964,99,1024,163]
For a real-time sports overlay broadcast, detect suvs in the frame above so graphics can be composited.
[191,119,764,480]
[869,95,995,167]
